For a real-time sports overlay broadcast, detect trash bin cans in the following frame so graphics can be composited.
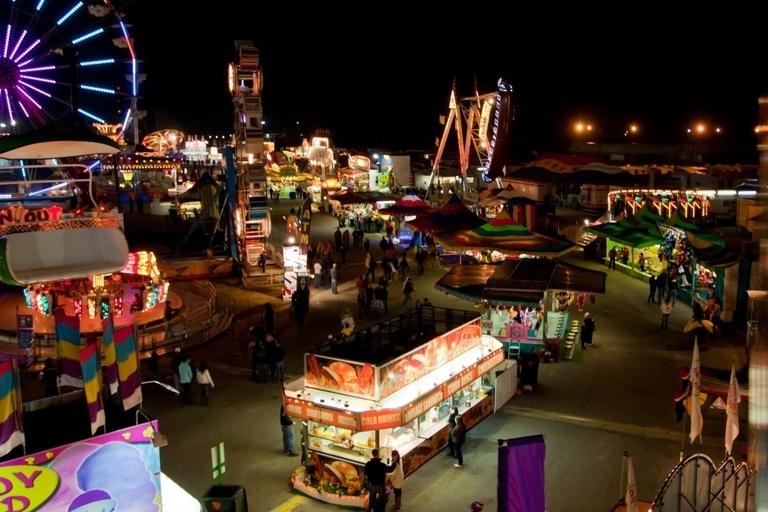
[203,484,249,512]
[519,351,541,384]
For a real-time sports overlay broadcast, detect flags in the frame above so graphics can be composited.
[689,338,703,445]
[725,364,741,455]
[0,358,26,457]
[54,312,143,436]
[625,456,639,512]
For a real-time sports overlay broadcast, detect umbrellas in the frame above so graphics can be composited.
[405,182,578,264]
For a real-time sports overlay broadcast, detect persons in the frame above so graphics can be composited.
[164,301,179,338]
[292,278,309,328]
[171,348,196,405]
[364,449,404,512]
[130,293,142,313]
[341,312,355,337]
[660,298,672,329]
[609,247,616,269]
[580,312,595,349]
[265,303,274,332]
[657,229,703,279]
[196,361,215,405]
[447,408,466,468]
[281,404,298,456]
[269,184,442,321]
[639,253,644,271]
[648,272,678,304]
[300,421,308,464]
[258,251,267,273]
[147,353,166,380]
[248,326,284,383]
[622,246,629,264]
[692,285,720,325]
[42,359,58,397]
